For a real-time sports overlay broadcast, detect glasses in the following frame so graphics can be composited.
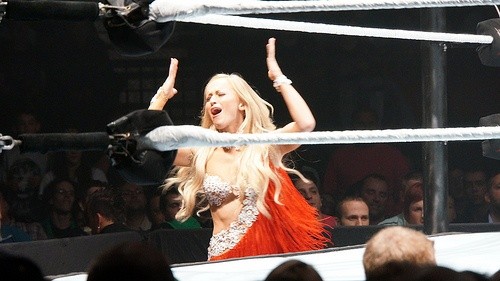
[56,189,73,195]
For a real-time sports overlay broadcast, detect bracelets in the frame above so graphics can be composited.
[272,75,292,90]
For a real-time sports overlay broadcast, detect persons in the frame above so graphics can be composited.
[147,37,334,261]
[0,111,500,281]
